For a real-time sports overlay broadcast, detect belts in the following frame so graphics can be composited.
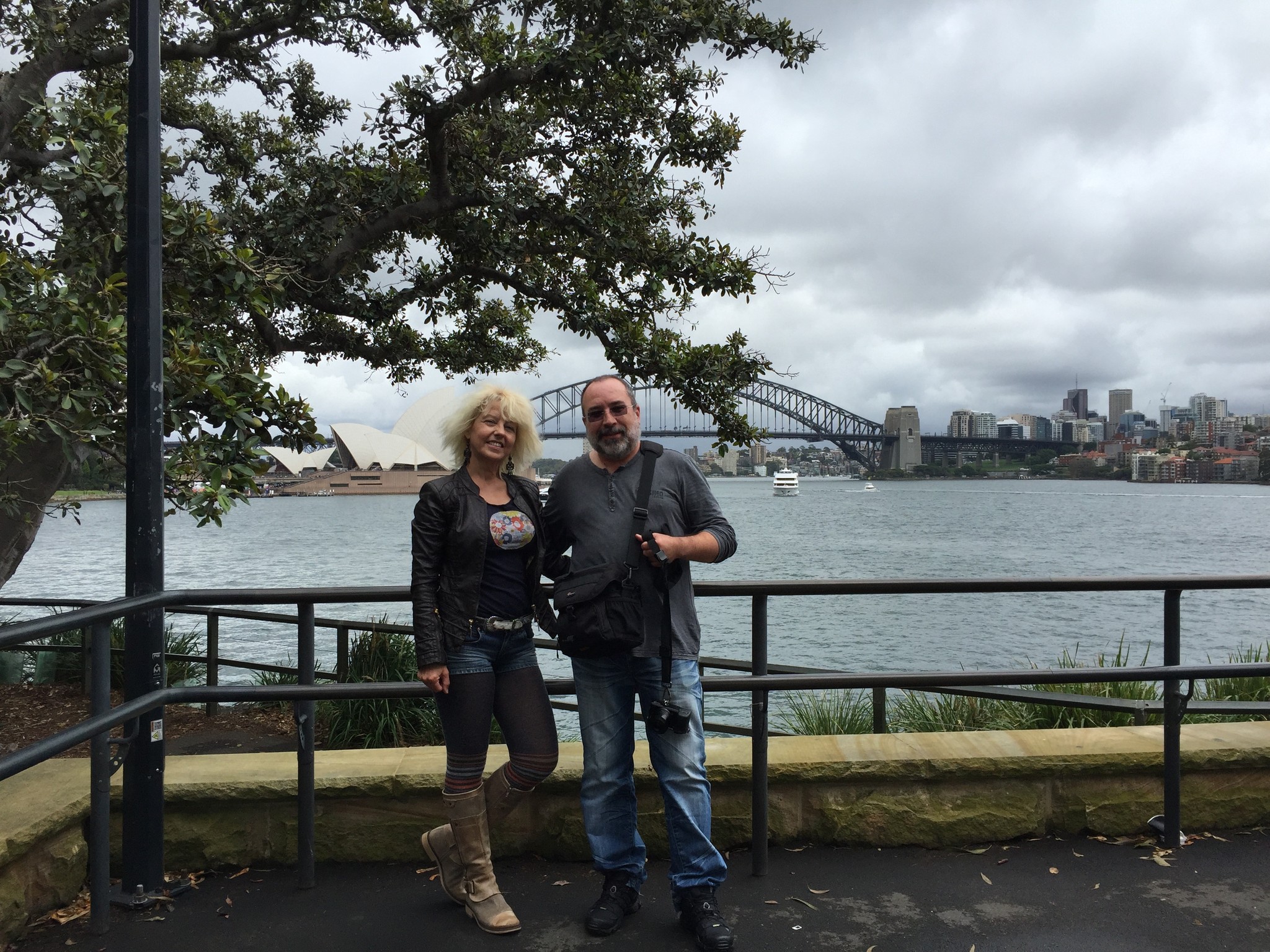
[473,615,537,633]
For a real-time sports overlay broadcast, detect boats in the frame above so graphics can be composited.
[773,458,800,496]
[864,483,878,491]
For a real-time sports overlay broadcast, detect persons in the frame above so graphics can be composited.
[411,390,570,935]
[539,376,739,951]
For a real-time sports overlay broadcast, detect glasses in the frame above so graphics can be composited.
[582,402,638,423]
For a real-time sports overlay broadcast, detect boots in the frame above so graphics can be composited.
[421,763,535,906]
[442,782,522,934]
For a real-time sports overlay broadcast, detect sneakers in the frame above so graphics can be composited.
[678,890,732,950]
[584,880,638,937]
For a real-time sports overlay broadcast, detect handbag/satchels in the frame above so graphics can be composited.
[552,564,646,658]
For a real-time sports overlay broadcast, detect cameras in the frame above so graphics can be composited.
[646,700,692,735]
